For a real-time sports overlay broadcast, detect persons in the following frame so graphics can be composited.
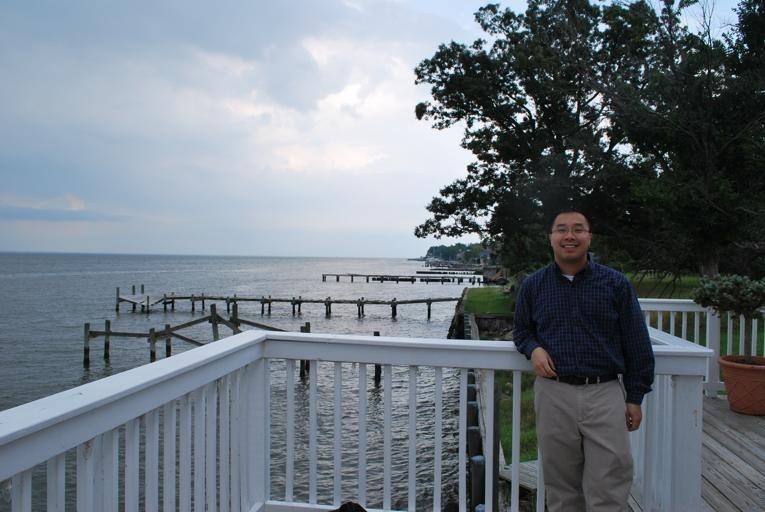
[512,207,654,511]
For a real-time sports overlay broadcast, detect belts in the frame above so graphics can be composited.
[542,376,618,387]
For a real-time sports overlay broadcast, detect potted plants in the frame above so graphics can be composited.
[692,274,765,416]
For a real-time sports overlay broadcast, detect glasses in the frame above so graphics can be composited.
[550,226,590,236]
